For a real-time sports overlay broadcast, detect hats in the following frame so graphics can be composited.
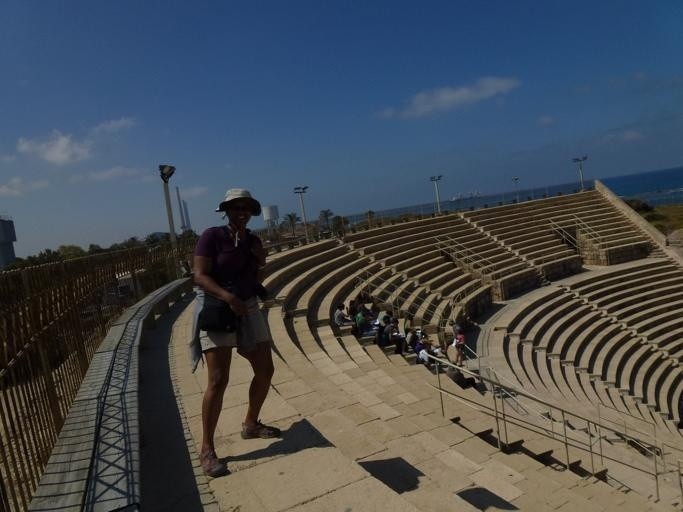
[214,187,261,217]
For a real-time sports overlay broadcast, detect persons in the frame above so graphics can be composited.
[333,302,479,392]
[191,187,282,478]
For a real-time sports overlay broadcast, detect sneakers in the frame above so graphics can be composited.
[199,450,227,477]
[240,421,280,439]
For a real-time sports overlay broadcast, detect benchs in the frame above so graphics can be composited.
[256,190,682,512]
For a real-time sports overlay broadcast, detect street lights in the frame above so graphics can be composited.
[512,176,522,202]
[573,155,588,189]
[294,186,310,242]
[430,174,442,214]
[159,164,182,278]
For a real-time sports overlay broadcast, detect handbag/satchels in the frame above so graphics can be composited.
[198,299,236,333]
